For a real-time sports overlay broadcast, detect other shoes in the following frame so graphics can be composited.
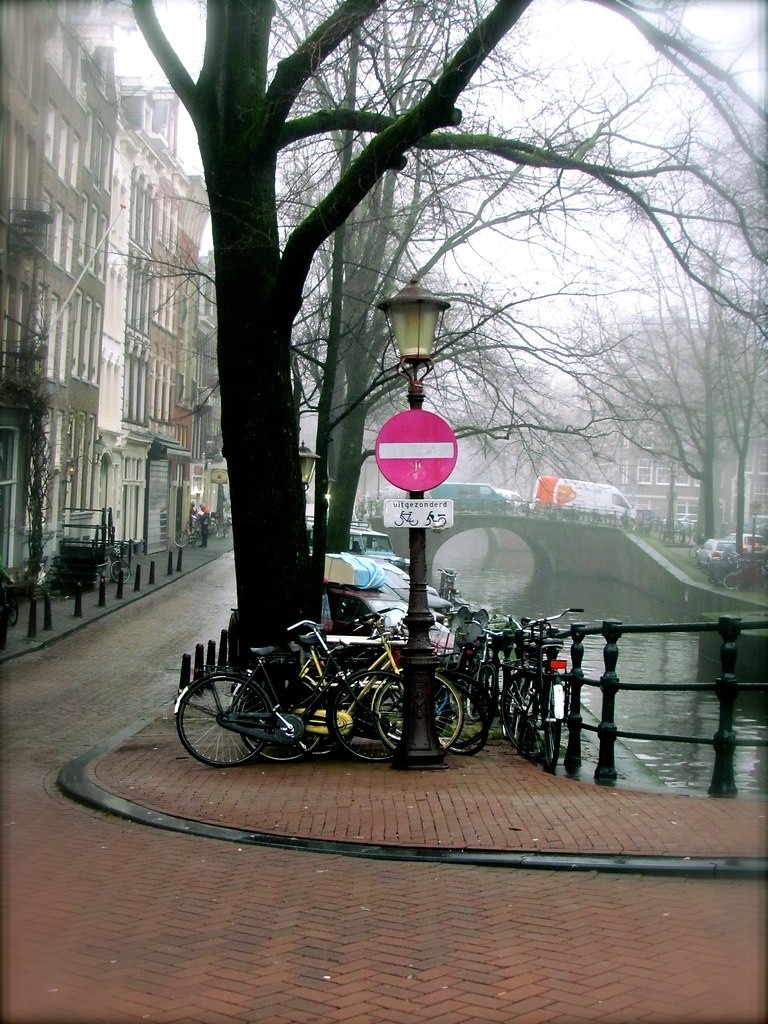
[199,544,207,548]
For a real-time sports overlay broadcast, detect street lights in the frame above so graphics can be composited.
[752,514,757,560]
[297,438,321,512]
[375,271,451,771]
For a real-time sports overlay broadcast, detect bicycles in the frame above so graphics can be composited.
[689,536,705,558]
[0,575,20,628]
[210,511,232,538]
[658,527,691,547]
[708,549,768,591]
[174,520,202,548]
[108,543,131,584]
[172,600,585,775]
[355,495,375,522]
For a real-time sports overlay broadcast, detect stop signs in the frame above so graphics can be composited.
[376,410,458,494]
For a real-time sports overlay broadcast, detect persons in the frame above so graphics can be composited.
[199,503,211,548]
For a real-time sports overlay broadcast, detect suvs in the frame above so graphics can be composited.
[722,532,768,555]
[661,512,698,531]
[347,527,411,571]
[323,586,451,640]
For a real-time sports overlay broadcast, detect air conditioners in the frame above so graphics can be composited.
[198,387,214,407]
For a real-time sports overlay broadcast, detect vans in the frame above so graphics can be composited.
[429,482,512,517]
[531,475,637,531]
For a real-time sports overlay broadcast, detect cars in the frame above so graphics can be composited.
[363,557,440,596]
[494,487,532,515]
[696,538,748,569]
[378,567,454,615]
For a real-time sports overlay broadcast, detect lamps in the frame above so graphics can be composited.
[75,435,105,464]
[60,459,75,485]
